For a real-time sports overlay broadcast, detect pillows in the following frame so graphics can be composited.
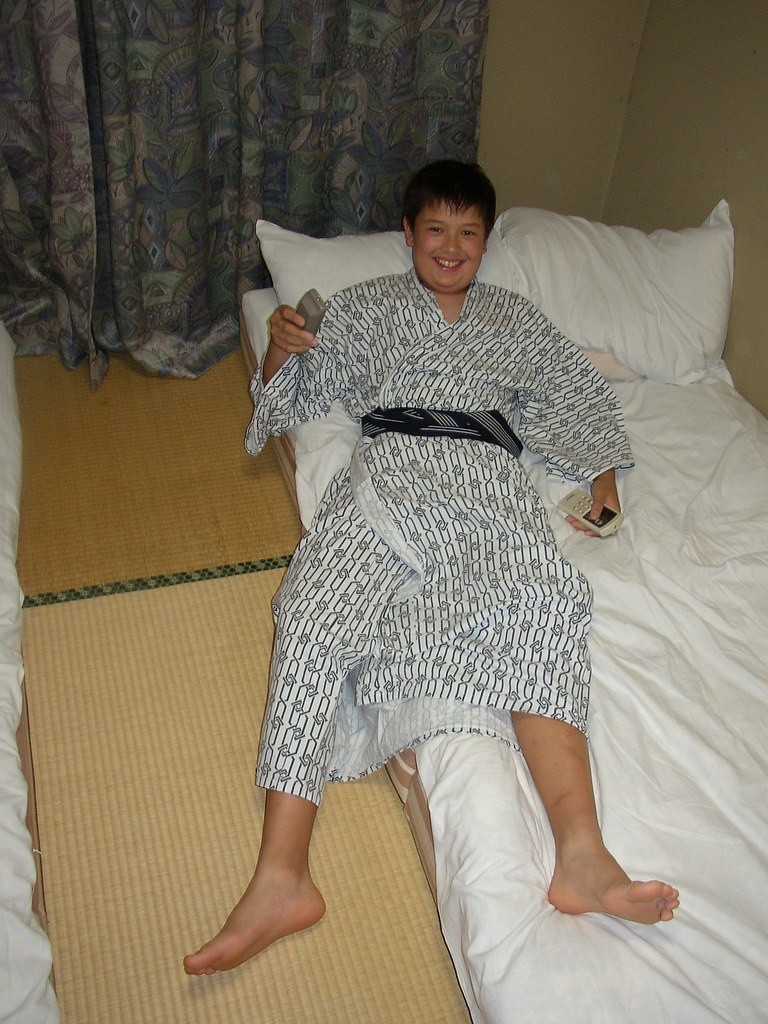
[493,198,739,388]
[255,219,529,308]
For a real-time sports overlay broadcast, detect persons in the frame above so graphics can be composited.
[184,157,679,978]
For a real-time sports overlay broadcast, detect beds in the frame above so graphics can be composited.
[239,288,768,1024]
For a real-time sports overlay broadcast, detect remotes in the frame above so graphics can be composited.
[294,288,328,336]
[556,486,625,537]
